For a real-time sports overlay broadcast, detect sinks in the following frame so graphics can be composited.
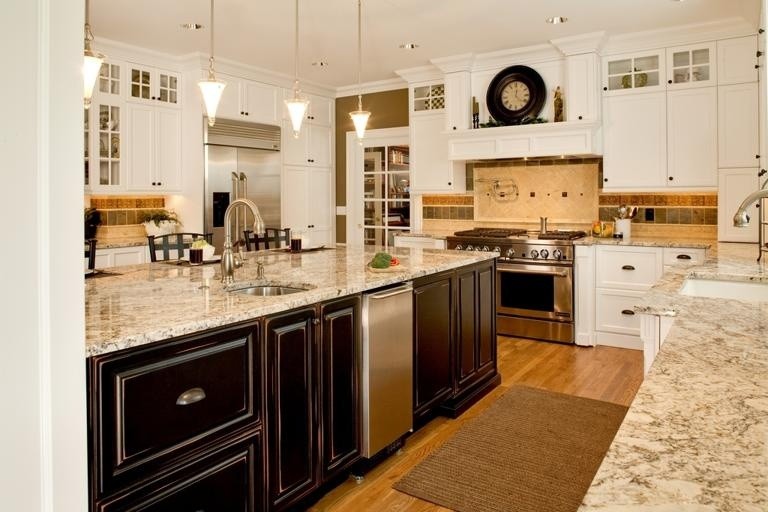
[678,278,768,299]
[224,282,319,297]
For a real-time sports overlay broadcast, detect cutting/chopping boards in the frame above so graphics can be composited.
[366,263,406,272]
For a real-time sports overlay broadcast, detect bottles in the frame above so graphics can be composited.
[554,86,563,122]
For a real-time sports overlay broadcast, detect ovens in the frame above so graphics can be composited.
[494,261,575,345]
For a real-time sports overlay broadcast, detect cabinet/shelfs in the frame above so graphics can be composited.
[85,317,263,512]
[83,100,126,194]
[601,84,716,192]
[717,3,765,244]
[411,257,502,419]
[574,244,709,352]
[283,126,331,164]
[96,61,123,95]
[284,89,331,125]
[126,106,182,194]
[607,40,718,88]
[128,64,179,106]
[260,295,360,512]
[411,80,465,193]
[203,72,275,123]
[280,167,331,250]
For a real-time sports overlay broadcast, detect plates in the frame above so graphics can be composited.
[621,70,648,88]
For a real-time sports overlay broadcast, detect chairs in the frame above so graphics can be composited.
[84,241,101,273]
[243,228,291,252]
[147,233,213,262]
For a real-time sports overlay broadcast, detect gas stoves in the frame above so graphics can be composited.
[447,227,585,267]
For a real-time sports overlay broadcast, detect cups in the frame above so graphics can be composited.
[684,67,700,82]
[615,218,632,238]
[675,74,684,84]
[188,238,203,265]
[290,230,302,253]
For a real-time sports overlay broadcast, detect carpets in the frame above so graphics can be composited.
[392,386,627,512]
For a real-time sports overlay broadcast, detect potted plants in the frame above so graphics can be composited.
[141,212,184,239]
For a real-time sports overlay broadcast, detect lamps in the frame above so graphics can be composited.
[83,1,107,110]
[347,0,373,145]
[283,0,311,139]
[195,0,227,127]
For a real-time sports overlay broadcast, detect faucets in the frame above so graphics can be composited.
[220,198,266,285]
[733,189,768,263]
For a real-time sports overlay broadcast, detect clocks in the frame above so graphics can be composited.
[485,65,545,123]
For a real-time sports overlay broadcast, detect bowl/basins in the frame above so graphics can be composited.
[592,221,614,238]
